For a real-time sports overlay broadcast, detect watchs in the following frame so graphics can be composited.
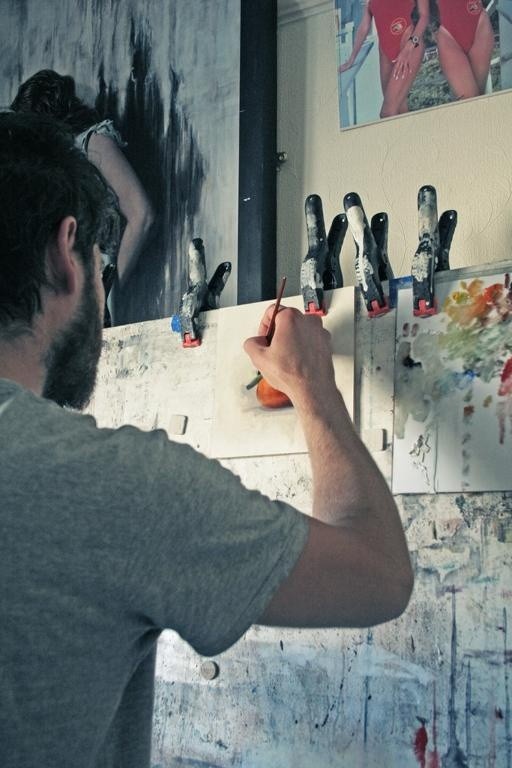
[406,34,420,48]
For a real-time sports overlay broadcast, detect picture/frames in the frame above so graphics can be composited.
[0,1,279,305]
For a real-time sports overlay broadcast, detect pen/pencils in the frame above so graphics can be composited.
[258,276,286,376]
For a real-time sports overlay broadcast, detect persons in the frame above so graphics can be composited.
[12,62,159,330]
[1,106,414,768]
[423,0,500,104]
[337,0,433,123]
